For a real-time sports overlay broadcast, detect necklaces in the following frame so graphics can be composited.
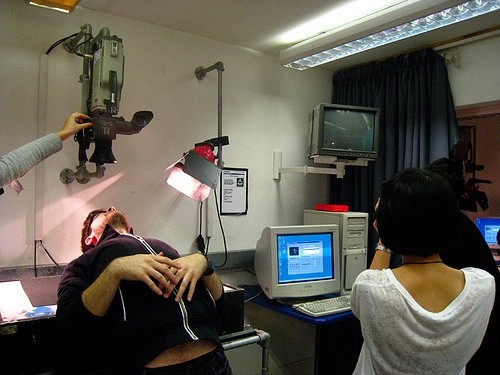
[400,261,444,265]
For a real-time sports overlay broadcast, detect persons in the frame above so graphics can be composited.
[54,205,233,375]
[350,178,500,375]
[0,111,93,189]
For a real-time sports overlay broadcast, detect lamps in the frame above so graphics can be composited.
[280,0,500,72]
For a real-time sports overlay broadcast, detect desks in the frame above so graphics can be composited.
[236,284,353,324]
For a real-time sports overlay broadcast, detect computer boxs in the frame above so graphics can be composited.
[303,209,369,296]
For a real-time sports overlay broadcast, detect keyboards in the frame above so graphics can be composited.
[292,295,351,317]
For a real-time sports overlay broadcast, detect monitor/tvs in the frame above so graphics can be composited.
[310,104,380,159]
[254,225,340,306]
[475,217,500,255]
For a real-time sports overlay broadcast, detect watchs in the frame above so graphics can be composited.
[374,242,394,255]
[202,258,214,278]
